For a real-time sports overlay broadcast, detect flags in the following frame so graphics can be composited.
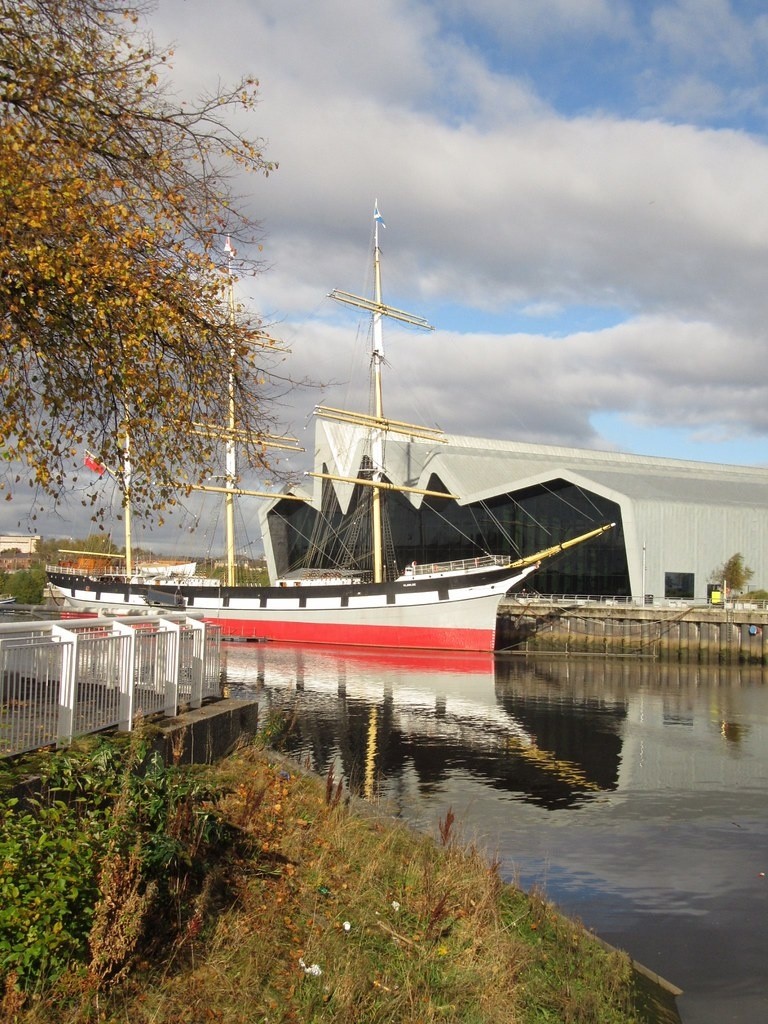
[84,451,104,474]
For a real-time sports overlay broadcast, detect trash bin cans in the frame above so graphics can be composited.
[645,595,653,605]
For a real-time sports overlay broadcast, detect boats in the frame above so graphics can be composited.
[137,560,198,578]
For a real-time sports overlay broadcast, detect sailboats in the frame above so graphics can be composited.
[54,619,603,843]
[42,193,619,656]
